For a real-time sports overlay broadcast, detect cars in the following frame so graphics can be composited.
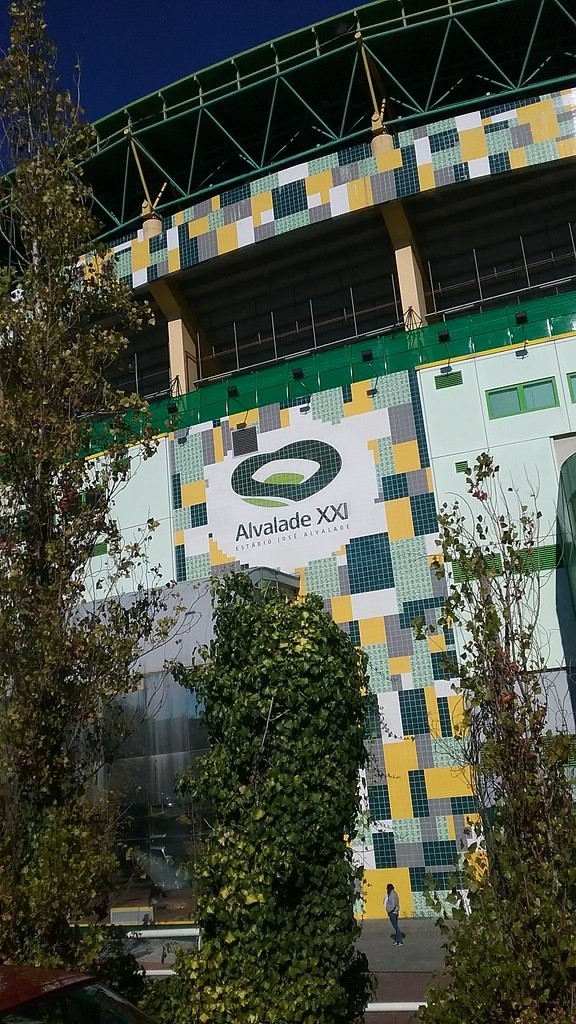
[0,966,159,1024]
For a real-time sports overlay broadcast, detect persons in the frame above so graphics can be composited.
[383,884,405,947]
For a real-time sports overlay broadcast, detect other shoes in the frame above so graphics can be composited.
[393,942,404,947]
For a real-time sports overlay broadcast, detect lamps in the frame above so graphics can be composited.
[515,311,528,325]
[227,385,239,397]
[438,330,449,342]
[361,349,373,362]
[292,367,305,380]
[167,404,179,414]
